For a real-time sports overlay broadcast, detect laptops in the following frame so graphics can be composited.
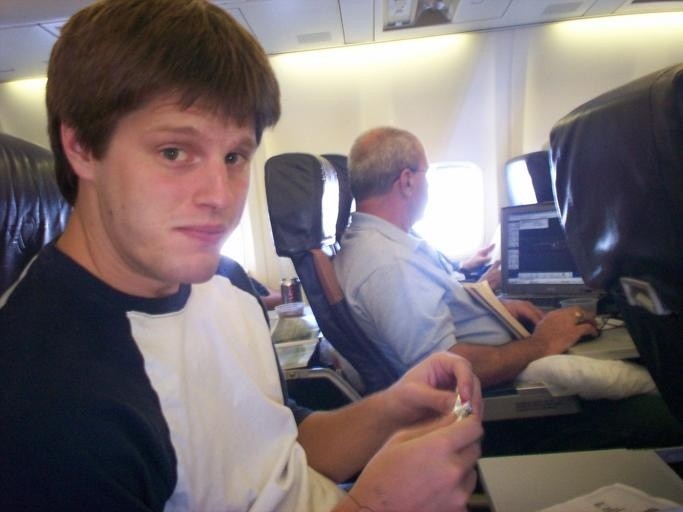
[489,202,605,322]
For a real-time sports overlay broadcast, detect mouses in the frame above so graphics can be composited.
[575,320,602,343]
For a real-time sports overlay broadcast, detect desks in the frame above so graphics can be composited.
[566,317,642,364]
[257,303,320,371]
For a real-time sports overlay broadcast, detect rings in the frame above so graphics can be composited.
[573,311,584,325]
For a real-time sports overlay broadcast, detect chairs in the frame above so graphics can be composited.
[279,364,362,413]
[0,133,78,309]
[545,64,682,430]
[502,151,553,209]
[323,154,356,245]
[262,151,577,456]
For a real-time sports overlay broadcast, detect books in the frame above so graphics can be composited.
[267,316,322,369]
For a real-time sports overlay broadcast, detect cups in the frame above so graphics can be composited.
[557,295,599,315]
[274,302,305,316]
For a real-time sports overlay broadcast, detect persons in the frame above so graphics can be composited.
[407,227,504,292]
[331,127,662,401]
[241,272,367,398]
[0,0,490,512]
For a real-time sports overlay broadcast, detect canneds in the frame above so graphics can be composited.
[280,277,303,304]
